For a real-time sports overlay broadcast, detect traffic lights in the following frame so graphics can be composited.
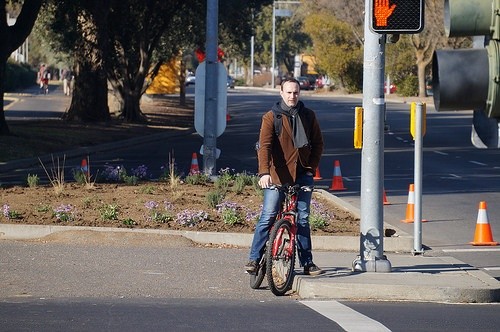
[370,0,425,33]
[432,2,500,126]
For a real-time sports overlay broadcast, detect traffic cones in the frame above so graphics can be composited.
[189,152,200,174]
[383,189,392,206]
[400,185,429,224]
[465,201,499,247]
[80,159,89,175]
[313,166,322,180]
[328,160,348,191]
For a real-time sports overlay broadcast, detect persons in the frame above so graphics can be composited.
[62,66,74,96]
[38,62,49,95]
[244,78,323,275]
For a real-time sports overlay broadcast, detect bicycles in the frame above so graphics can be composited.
[247,183,319,295]
[42,79,48,93]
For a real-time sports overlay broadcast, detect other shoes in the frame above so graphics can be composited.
[304,262,323,276]
[245,260,259,276]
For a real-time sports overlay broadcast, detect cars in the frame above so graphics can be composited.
[185,73,197,85]
[226,76,235,88]
[297,73,327,91]
